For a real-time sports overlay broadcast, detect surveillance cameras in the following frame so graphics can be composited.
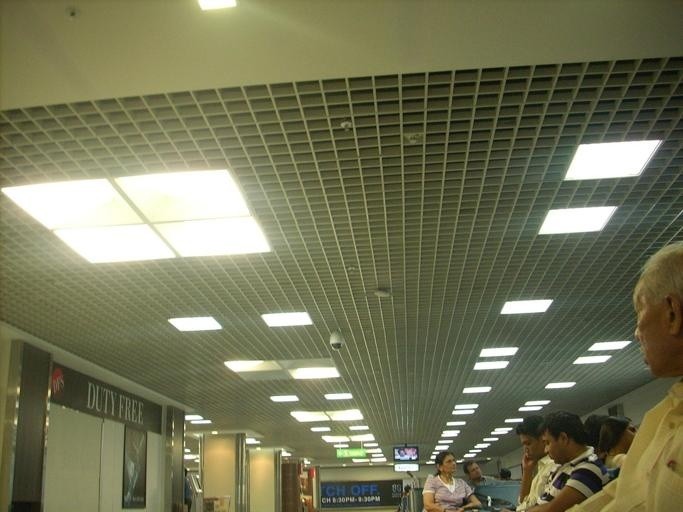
[329,331,345,351]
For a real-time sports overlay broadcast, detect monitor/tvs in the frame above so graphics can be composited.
[393,446,419,461]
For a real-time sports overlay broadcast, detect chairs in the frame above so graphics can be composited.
[407,480,523,512]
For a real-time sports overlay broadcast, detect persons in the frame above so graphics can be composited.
[421,452,481,512]
[463,460,499,486]
[398,448,418,460]
[563,241,682,511]
[515,411,638,512]
[185,468,192,512]
[500,468,511,479]
